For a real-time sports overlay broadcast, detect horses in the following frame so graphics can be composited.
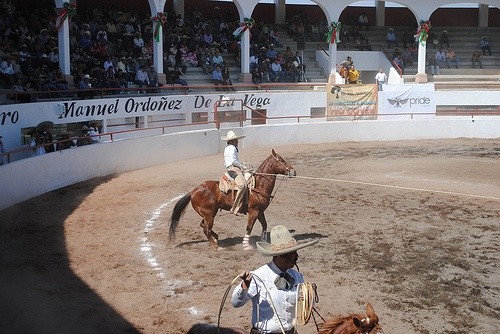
[187,301,385,334]
[168,149,297,250]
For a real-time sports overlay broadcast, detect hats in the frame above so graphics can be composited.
[220,131,245,141]
[257,225,319,256]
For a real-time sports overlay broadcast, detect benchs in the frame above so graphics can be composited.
[160,27,500,93]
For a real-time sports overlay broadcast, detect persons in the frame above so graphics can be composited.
[472,51,483,69]
[249,17,306,84]
[0,141,5,165]
[68,8,163,100]
[481,35,492,56]
[441,28,449,48]
[386,27,409,74]
[163,7,241,91]
[221,130,251,216]
[338,56,360,84]
[407,30,437,48]
[435,48,459,68]
[0,0,67,102]
[231,221,320,334]
[375,67,387,91]
[286,10,369,51]
[31,119,103,155]
